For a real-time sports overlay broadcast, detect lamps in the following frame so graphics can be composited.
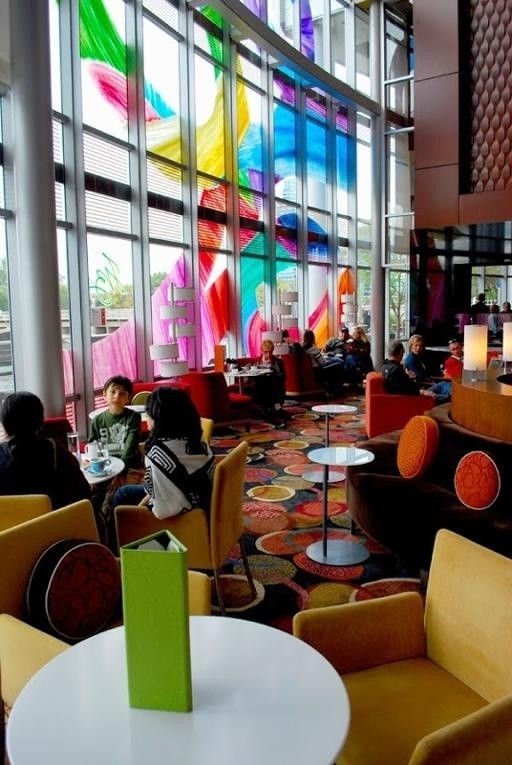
[502,322,511,366]
[339,292,356,326]
[149,281,196,383]
[261,290,301,355]
[462,324,488,382]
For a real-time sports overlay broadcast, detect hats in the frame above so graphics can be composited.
[337,325,349,333]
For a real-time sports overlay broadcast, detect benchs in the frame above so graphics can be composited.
[128,352,331,431]
[344,401,510,572]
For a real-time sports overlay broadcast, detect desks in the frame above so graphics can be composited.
[5,615,351,764]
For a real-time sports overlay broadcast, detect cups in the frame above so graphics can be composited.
[98,449,109,463]
[251,366,257,374]
[231,368,238,373]
[67,432,81,464]
[89,458,112,473]
[84,442,97,460]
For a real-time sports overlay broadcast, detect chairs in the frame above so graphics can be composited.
[293,528,511,763]
[2,390,257,707]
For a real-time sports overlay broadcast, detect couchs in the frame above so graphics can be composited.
[365,372,436,439]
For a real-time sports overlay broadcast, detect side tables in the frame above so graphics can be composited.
[307,446,375,566]
[302,404,358,483]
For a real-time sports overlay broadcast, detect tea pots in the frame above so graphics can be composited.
[225,359,240,373]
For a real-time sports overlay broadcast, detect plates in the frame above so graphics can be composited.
[87,468,112,477]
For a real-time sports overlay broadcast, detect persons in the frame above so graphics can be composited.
[252,340,291,424]
[107,386,216,556]
[0,390,91,510]
[290,293,512,407]
[87,376,147,526]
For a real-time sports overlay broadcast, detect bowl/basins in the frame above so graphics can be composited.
[241,367,249,371]
